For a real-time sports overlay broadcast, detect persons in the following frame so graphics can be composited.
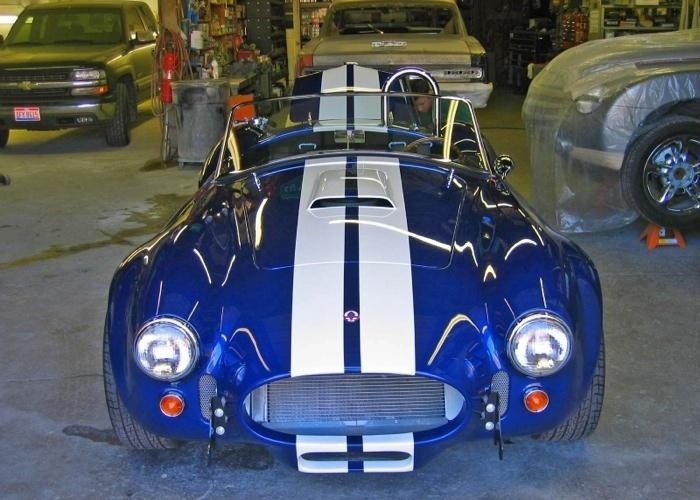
[409,79,473,132]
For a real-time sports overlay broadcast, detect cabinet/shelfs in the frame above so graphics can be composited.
[596,1,689,39]
[158,0,290,171]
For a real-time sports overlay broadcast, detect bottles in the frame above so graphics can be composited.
[199,59,221,79]
[257,54,281,72]
[562,12,588,43]
[180,0,237,65]
[299,0,320,39]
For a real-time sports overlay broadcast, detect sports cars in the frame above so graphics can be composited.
[100,56,611,480]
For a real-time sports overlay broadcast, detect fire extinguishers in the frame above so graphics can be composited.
[161,40,176,103]
